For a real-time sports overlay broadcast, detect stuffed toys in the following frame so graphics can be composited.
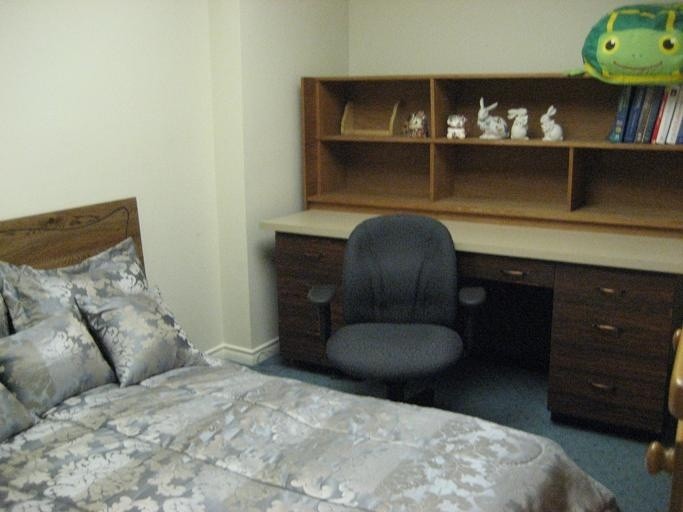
[565,1,683,86]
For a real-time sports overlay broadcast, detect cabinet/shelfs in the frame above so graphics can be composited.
[298,72,683,231]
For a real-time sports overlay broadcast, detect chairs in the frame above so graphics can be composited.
[306,213,488,408]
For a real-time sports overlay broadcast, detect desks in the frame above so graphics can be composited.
[259,208,682,445]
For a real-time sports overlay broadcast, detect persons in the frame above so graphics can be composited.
[608,84,683,145]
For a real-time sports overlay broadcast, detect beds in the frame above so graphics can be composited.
[0,195,618,512]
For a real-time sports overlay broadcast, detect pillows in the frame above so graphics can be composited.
[75,283,213,388]
[2,238,149,351]
[1,308,119,416]
[1,384,39,441]
